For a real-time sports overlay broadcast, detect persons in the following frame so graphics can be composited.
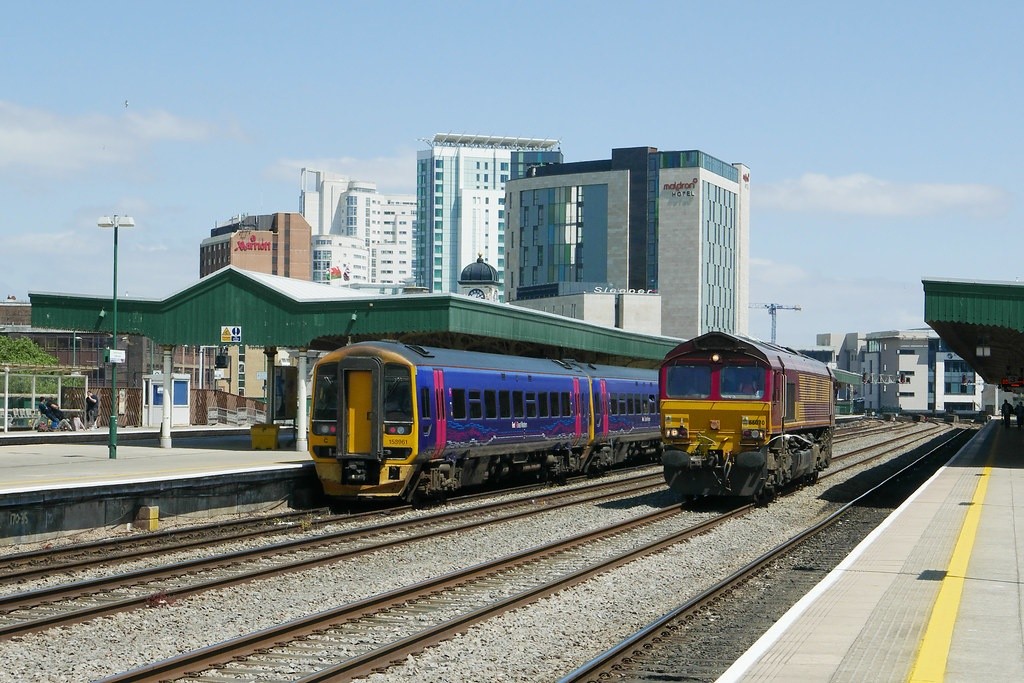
[1014,401,1024,431]
[86,390,99,422]
[1001,399,1013,429]
[57,413,86,431]
[39,394,80,424]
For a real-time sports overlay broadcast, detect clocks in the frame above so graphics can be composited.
[468,289,485,299]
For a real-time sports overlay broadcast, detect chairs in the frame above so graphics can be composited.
[13,408,39,430]
[0,408,4,418]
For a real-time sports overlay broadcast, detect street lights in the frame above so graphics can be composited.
[97,213,136,461]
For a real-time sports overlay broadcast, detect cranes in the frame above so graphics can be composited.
[750,302,803,347]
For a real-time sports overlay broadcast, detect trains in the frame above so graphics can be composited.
[306,337,660,510]
[658,331,839,505]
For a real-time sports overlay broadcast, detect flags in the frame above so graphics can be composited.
[343,262,351,281]
[326,265,342,280]
